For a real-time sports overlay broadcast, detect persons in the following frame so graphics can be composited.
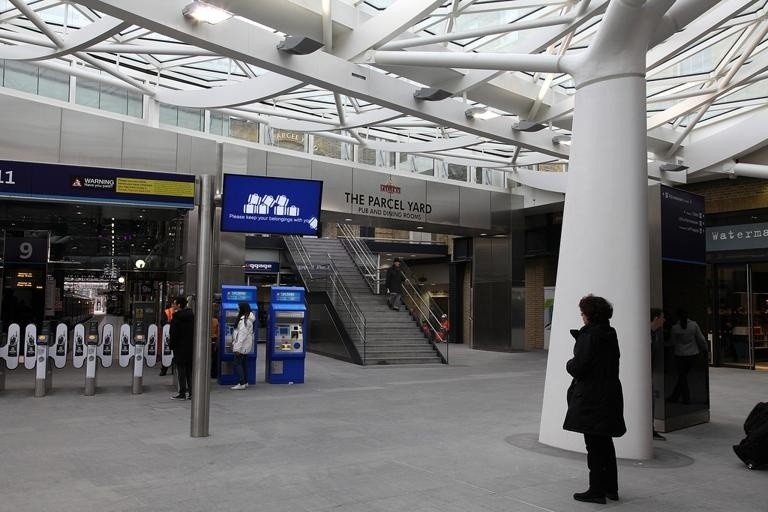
[384,257,410,311]
[563,292,628,505]
[230,301,257,391]
[648,307,667,441]
[168,296,195,400]
[668,308,709,406]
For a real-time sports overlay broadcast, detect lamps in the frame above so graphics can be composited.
[412,87,454,102]
[466,107,549,132]
[276,35,326,55]
[182,0,236,25]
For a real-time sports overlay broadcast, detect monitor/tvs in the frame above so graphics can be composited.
[275,325,290,337]
[226,325,234,336]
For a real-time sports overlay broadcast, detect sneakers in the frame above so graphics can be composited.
[186,392,192,400]
[652,430,665,440]
[170,393,186,401]
[393,306,399,311]
[230,383,245,390]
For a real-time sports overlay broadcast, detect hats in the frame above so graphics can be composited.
[394,258,401,262]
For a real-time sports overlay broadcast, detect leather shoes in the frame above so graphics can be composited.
[606,492,619,501]
[160,370,166,376]
[572,488,606,504]
